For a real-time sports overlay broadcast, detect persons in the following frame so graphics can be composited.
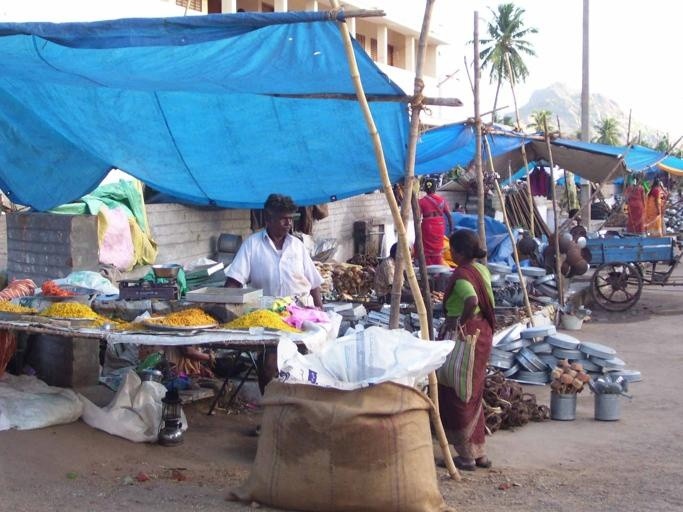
[625,176,667,236]
[219,194,323,436]
[375,242,423,304]
[431,228,496,470]
[419,179,453,266]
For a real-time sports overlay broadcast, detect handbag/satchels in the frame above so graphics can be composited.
[435,331,476,403]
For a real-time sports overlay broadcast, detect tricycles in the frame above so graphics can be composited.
[514,229,676,312]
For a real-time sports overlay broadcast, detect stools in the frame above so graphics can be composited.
[206,347,263,415]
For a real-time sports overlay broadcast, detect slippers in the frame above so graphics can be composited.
[440,456,491,470]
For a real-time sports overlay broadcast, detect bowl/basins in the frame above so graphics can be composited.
[151,264,181,277]
[329,262,643,386]
[139,368,163,383]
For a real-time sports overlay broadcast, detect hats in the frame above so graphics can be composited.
[264,194,296,213]
